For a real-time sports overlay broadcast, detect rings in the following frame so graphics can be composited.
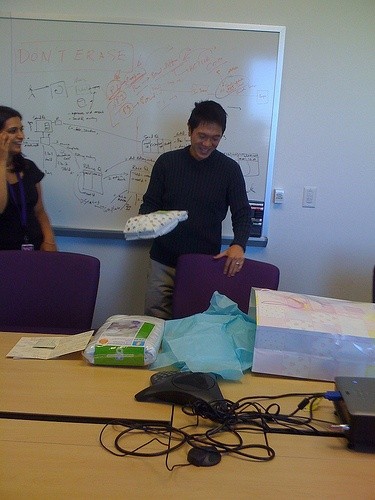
[236,262,240,265]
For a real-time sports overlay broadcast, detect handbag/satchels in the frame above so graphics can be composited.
[249,287,375,382]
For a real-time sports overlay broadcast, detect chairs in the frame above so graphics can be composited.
[170,254,280,321]
[0,250,100,336]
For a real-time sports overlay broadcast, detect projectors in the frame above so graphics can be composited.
[332,374,374,449]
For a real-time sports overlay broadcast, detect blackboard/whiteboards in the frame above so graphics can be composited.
[0,11,286,247]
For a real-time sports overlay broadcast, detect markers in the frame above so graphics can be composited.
[251,223,262,225]
[249,203,263,206]
[251,218,262,222]
[250,207,263,210]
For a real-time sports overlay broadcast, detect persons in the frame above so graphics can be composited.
[139,100,253,319]
[0,105,56,252]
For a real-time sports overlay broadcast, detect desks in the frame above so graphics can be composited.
[0,331,375,500]
